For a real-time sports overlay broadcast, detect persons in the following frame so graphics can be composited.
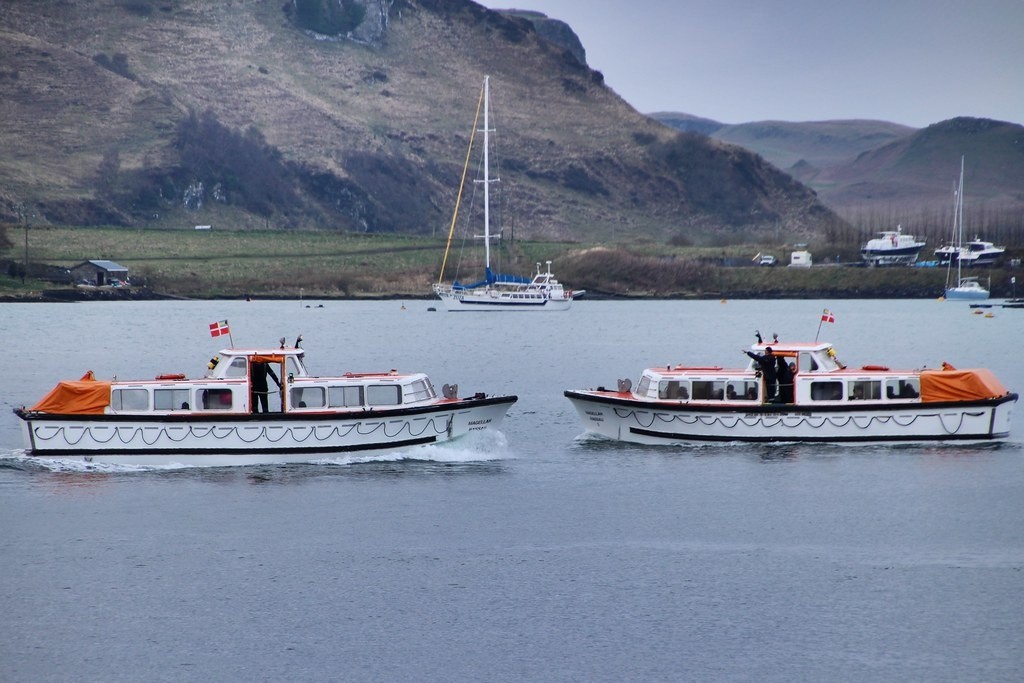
[677,387,689,399]
[853,385,863,399]
[887,386,895,397]
[902,383,915,396]
[251,362,281,413]
[775,356,788,369]
[780,362,796,403]
[741,346,777,398]
[748,390,756,399]
[719,385,736,400]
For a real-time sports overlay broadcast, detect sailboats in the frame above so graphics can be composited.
[432,77,573,311]
[943,155,989,300]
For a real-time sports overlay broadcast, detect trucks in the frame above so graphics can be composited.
[791,251,813,267]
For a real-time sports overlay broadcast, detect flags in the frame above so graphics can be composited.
[822,309,834,323]
[209,320,229,337]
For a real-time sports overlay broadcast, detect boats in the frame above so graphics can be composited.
[562,329,1018,448]
[572,290,586,299]
[11,335,518,464]
[859,224,1005,269]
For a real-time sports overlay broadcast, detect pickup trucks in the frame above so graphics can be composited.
[760,256,776,266]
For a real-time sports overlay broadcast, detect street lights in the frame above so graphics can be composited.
[20,211,35,274]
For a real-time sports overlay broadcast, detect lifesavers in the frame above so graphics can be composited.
[155,373,185,380]
[861,365,890,372]
